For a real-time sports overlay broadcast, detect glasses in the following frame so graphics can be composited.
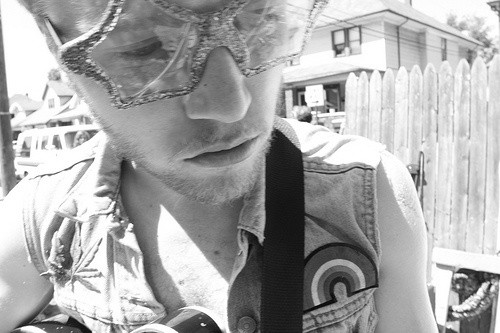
[38,0,329,109]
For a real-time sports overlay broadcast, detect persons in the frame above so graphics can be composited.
[1,0,439,333]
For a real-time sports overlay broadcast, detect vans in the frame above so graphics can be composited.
[13,125,101,182]
